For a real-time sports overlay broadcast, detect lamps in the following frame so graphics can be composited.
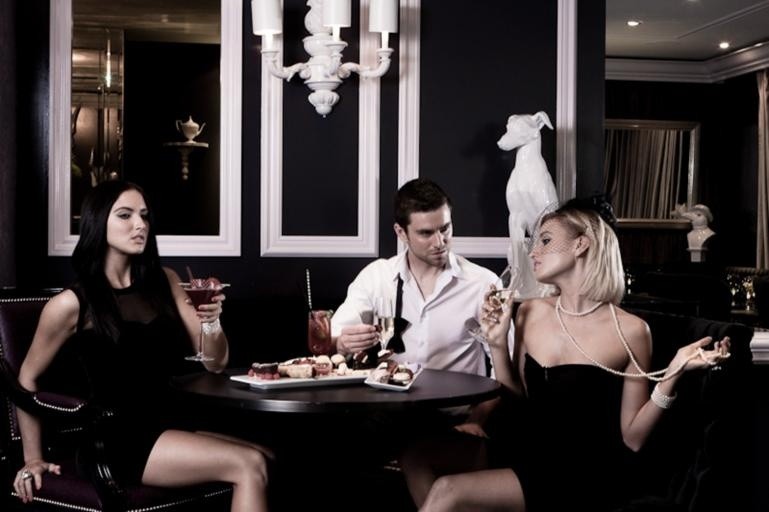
[252,2,397,117]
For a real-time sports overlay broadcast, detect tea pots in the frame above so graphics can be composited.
[176,115,205,143]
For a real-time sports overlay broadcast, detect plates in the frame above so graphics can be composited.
[229,368,364,388]
[364,361,424,390]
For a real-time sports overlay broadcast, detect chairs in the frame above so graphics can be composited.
[489,313,755,512]
[1,284,232,512]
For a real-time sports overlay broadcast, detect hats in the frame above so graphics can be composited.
[688,204,712,225]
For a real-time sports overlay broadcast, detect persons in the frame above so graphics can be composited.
[12,180,271,512]
[330,178,515,380]
[404,198,731,511]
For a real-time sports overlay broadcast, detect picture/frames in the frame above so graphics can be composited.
[258,5,381,258]
[398,0,577,260]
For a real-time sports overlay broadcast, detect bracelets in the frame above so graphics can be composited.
[650,383,678,409]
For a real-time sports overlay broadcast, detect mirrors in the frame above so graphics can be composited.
[49,0,243,256]
[604,119,698,228]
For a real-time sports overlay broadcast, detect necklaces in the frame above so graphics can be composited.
[554,295,730,382]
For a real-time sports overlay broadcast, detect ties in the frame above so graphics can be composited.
[387,279,411,355]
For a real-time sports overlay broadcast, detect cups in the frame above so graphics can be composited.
[469,266,522,346]
[179,282,228,361]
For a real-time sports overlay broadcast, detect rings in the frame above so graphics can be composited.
[21,469,32,480]
